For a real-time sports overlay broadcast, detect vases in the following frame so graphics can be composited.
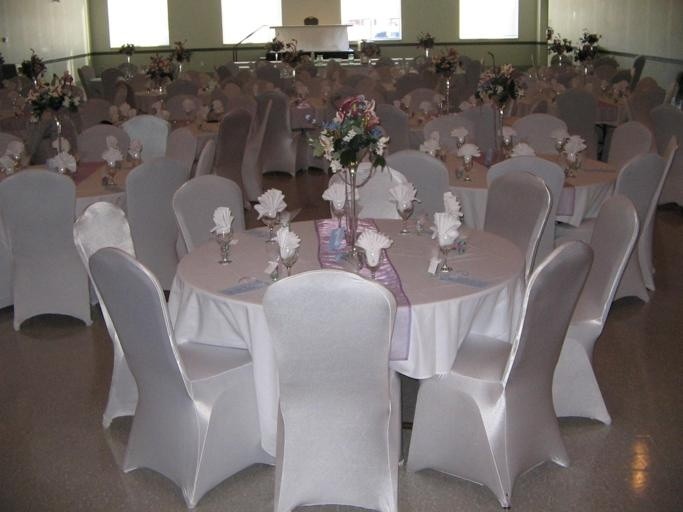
[341,168,365,269]
[445,79,450,115]
[499,110,504,140]
[53,112,65,172]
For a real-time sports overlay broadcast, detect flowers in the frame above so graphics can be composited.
[304,94,389,175]
[474,52,526,110]
[432,49,464,79]
[13,71,85,124]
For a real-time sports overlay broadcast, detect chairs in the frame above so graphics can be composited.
[120,115,170,162]
[166,127,197,177]
[405,241,594,509]
[483,170,554,287]
[0,211,14,308]
[511,113,567,155]
[241,99,273,201]
[77,125,130,162]
[558,89,598,159]
[328,163,408,219]
[73,201,138,429]
[385,150,448,220]
[442,56,482,111]
[167,95,201,120]
[556,153,666,303]
[308,130,331,174]
[119,63,136,72]
[375,104,410,154]
[639,135,679,291]
[423,116,472,157]
[630,54,646,92]
[486,155,565,273]
[628,77,666,122]
[608,121,658,171]
[176,54,436,91]
[165,80,199,100]
[649,105,683,207]
[193,139,216,177]
[172,175,246,254]
[212,89,230,112]
[223,82,240,97]
[0,170,93,330]
[552,195,639,425]
[224,92,257,139]
[263,269,400,512]
[401,89,442,110]
[212,108,252,210]
[101,67,125,98]
[88,247,275,508]
[125,158,189,290]
[257,91,308,178]
[77,66,95,100]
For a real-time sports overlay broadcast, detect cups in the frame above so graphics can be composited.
[104,158,117,185]
[566,152,580,178]
[554,138,566,161]
[329,200,345,228]
[56,166,65,174]
[456,137,465,156]
[500,136,512,158]
[396,200,414,234]
[130,149,139,166]
[279,248,299,277]
[12,152,21,172]
[364,251,382,280]
[462,157,473,182]
[215,227,233,264]
[438,233,454,273]
[425,150,439,159]
[260,210,279,244]
